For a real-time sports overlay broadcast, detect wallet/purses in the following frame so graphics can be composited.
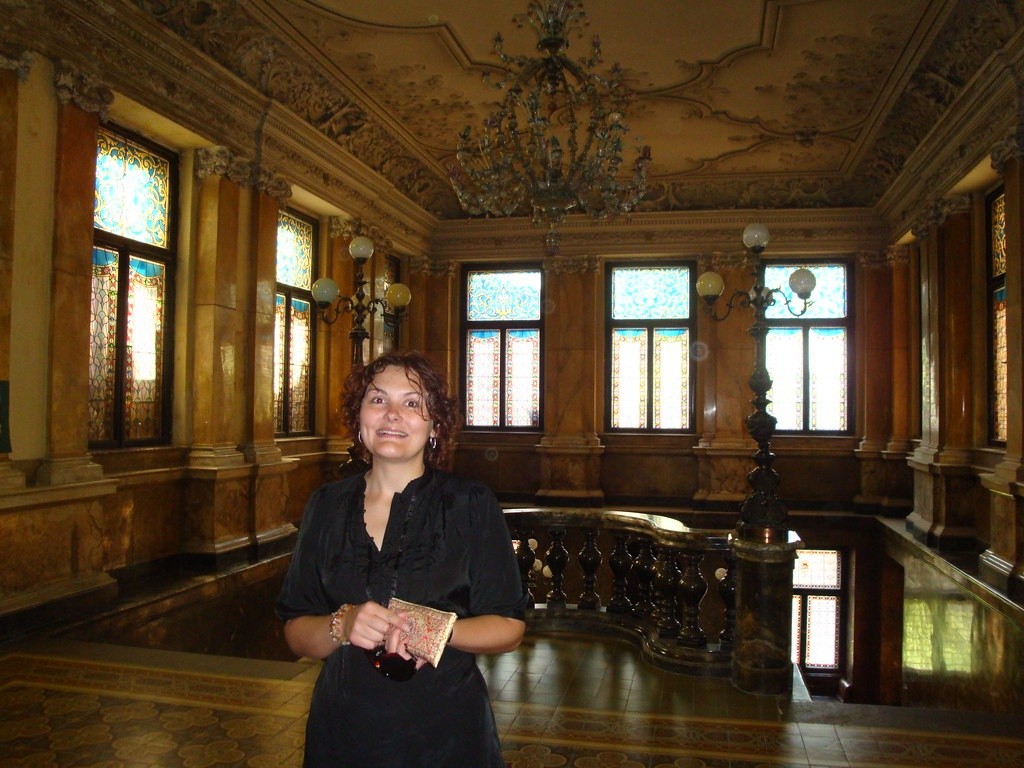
[387,597,458,669]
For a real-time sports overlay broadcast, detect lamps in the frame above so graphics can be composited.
[696,223,816,543]
[312,237,412,477]
[445,0,653,258]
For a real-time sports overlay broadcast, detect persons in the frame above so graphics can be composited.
[273,347,527,768]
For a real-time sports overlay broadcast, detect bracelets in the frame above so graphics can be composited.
[330,604,353,647]
